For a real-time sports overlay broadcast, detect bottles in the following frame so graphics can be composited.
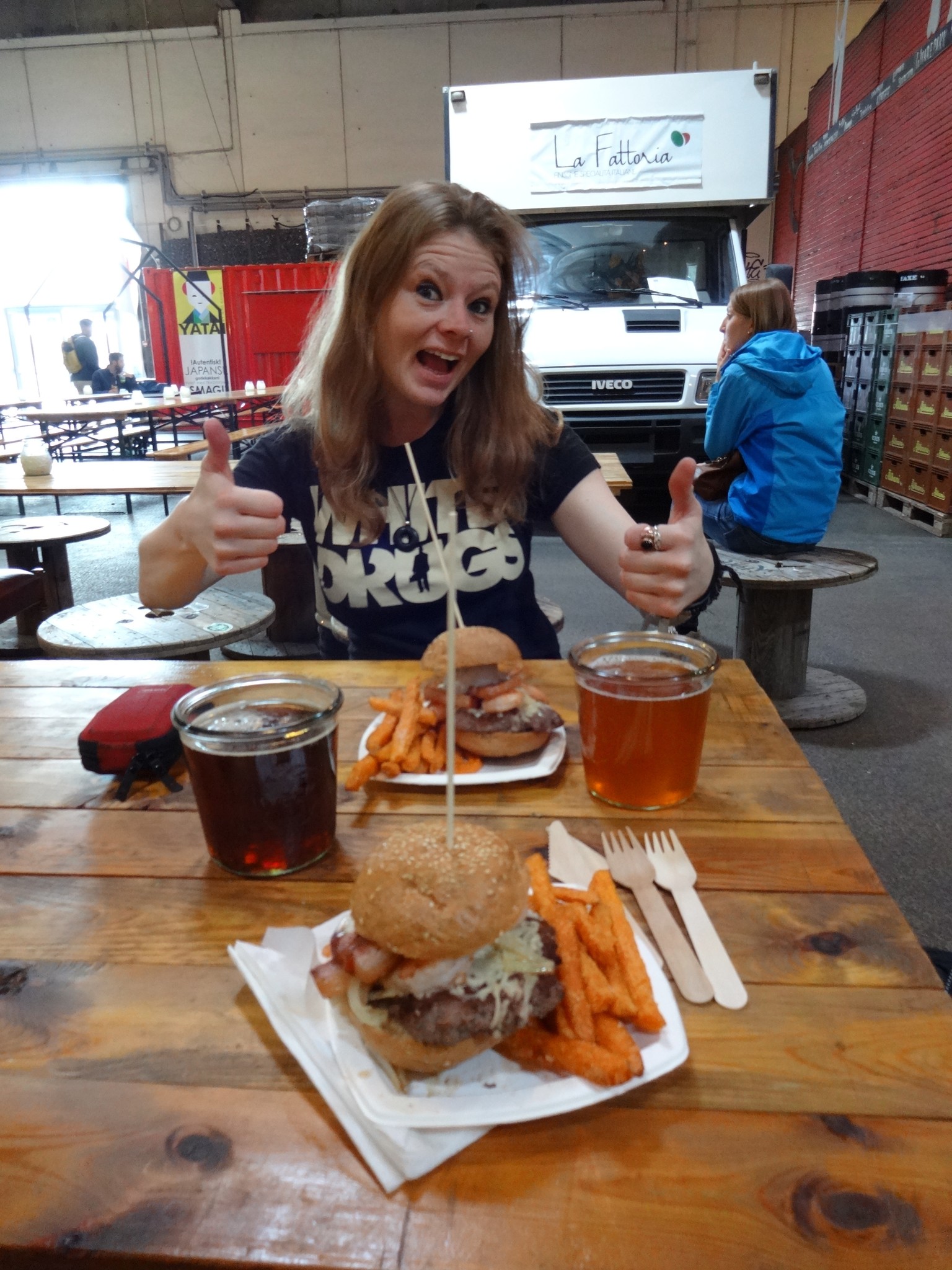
[171,385,178,394]
[132,390,144,404]
[245,381,254,391]
[256,381,266,390]
[119,389,127,398]
[21,439,52,476]
[180,386,191,397]
[83,385,93,395]
[164,387,175,399]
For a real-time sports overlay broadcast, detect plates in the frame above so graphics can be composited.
[358,701,567,786]
[326,882,690,1127]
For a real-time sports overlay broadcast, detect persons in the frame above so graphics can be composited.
[91,352,137,420]
[61,319,102,431]
[643,279,847,638]
[138,180,721,660]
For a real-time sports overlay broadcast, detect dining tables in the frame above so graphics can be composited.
[0,660,952,1270]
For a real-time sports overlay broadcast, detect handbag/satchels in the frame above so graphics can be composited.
[692,450,747,503]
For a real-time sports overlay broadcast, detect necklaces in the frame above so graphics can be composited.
[377,463,421,553]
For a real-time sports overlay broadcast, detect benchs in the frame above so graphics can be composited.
[145,423,283,461]
[58,426,162,461]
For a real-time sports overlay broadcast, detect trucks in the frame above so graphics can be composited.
[437,64,781,519]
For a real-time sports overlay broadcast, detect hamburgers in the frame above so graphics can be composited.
[311,820,562,1075]
[420,625,566,757]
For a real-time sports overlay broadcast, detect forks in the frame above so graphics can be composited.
[645,829,748,1010]
[601,826,714,1004]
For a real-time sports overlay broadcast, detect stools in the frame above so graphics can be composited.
[709,542,879,729]
[37,590,278,660]
[0,516,111,619]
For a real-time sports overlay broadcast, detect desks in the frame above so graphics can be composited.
[0,461,234,517]
[0,384,293,460]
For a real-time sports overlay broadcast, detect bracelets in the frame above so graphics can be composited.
[682,541,723,612]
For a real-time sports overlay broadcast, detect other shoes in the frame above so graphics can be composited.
[669,626,702,642]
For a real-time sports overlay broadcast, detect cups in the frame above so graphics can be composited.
[171,672,345,876]
[567,630,722,809]
[118,373,126,383]
[112,386,117,393]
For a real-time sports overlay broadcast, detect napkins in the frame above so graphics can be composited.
[228,910,692,1196]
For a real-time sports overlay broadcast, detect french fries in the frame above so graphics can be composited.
[345,674,484,791]
[493,851,667,1085]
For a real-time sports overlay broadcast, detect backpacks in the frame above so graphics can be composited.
[62,335,86,374]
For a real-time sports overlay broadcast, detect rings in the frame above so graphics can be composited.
[641,524,654,552]
[653,525,662,551]
